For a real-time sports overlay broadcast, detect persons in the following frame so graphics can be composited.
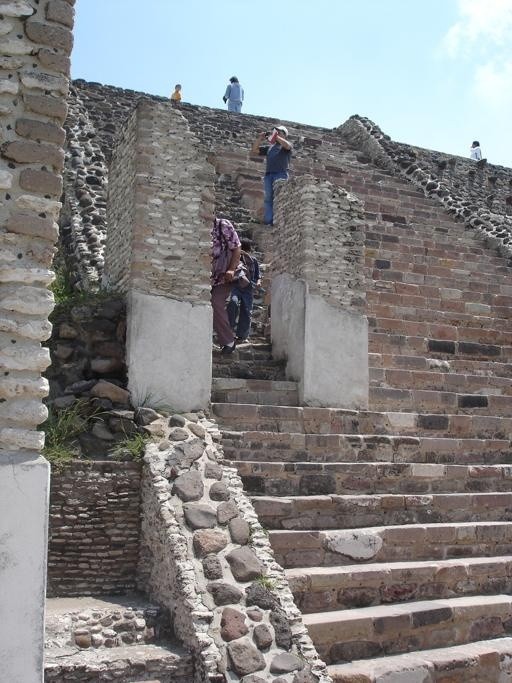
[253,124,294,224]
[222,76,245,112]
[467,140,482,160]
[212,212,240,353]
[228,239,262,343]
[170,82,182,100]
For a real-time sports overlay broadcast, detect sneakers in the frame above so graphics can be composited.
[235,337,249,344]
[222,342,235,353]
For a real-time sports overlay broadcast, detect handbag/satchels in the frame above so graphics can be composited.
[230,261,250,291]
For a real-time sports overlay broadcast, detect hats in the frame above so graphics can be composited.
[274,125,288,138]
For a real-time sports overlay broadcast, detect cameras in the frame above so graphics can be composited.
[265,132,272,139]
